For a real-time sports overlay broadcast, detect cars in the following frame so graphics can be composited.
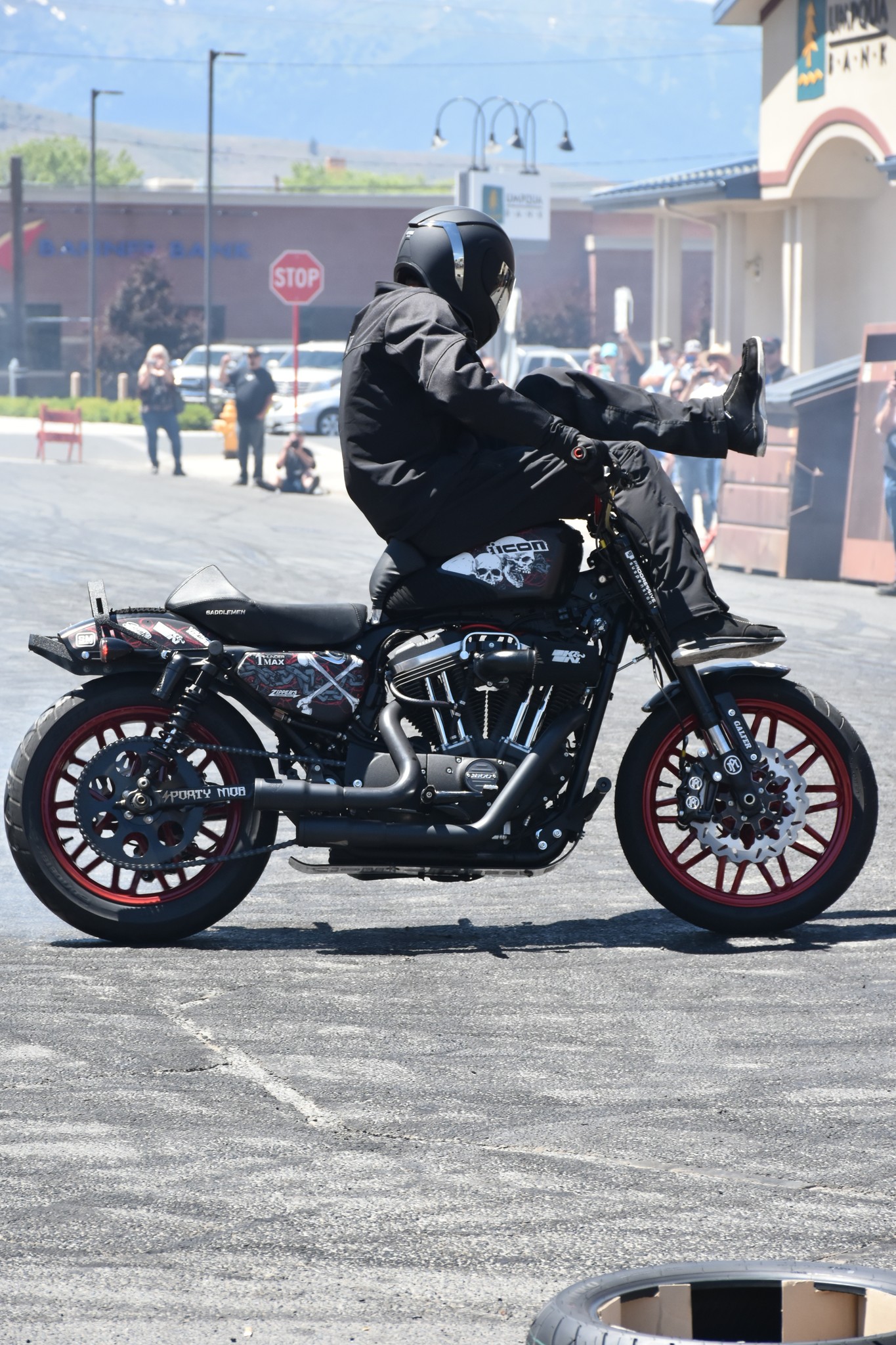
[165,340,346,438]
[500,343,582,393]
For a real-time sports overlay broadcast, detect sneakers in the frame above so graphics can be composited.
[727,336,769,457]
[670,615,787,666]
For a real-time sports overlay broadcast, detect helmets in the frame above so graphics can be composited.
[393,204,515,351]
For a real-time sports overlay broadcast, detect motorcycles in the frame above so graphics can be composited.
[0,445,879,952]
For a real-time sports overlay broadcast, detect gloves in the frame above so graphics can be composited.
[566,433,623,481]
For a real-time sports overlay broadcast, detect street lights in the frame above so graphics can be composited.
[202,50,249,411]
[87,90,127,397]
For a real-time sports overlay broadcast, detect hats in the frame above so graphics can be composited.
[602,342,616,359]
[684,338,701,355]
[701,342,736,368]
[240,345,260,357]
[763,337,782,353]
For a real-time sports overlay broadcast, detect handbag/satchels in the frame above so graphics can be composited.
[170,389,185,413]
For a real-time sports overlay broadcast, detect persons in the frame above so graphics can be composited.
[256,433,320,495]
[580,328,798,538]
[136,344,187,475]
[339,206,787,668]
[873,380,895,596]
[218,344,278,488]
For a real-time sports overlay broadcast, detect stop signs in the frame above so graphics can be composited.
[268,249,325,307]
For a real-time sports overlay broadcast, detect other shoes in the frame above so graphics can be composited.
[876,581,896,595]
[259,482,277,491]
[306,476,320,494]
